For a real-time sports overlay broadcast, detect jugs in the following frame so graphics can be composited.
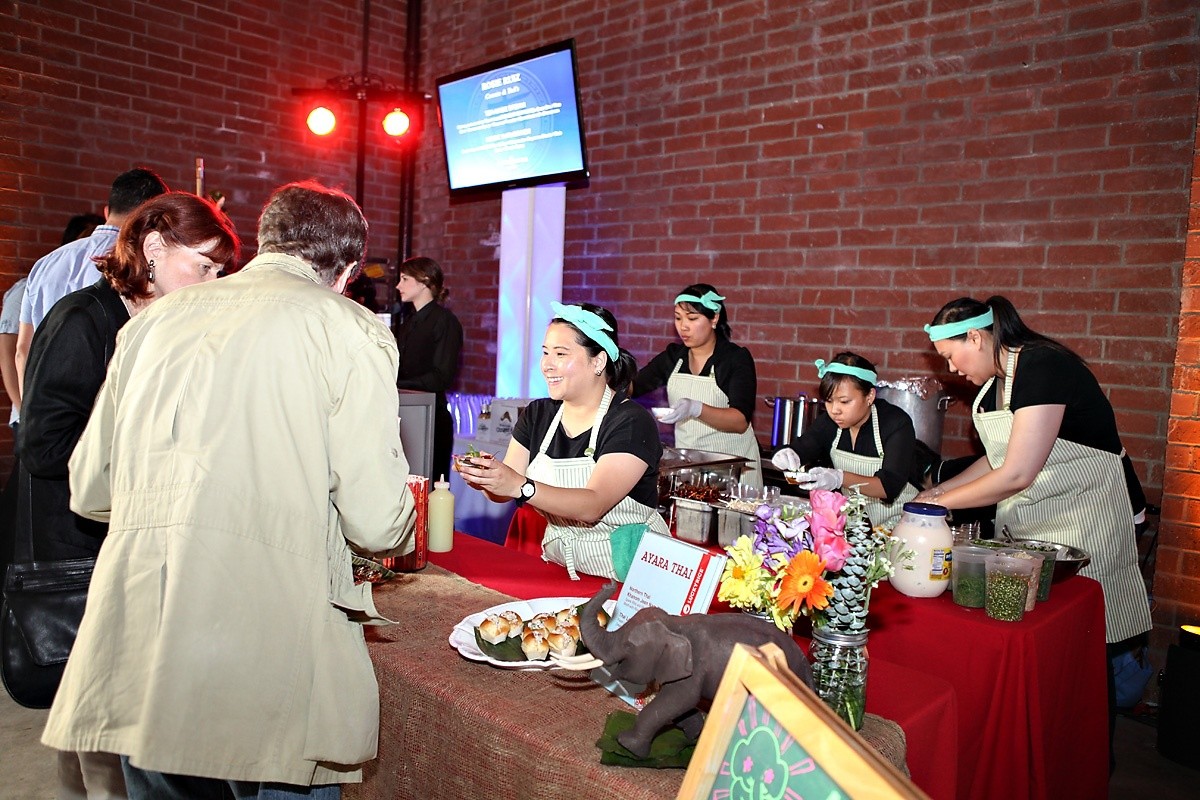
[381,475,428,573]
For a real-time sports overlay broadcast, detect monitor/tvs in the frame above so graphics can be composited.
[432,35,591,199]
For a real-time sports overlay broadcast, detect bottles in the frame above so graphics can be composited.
[946,522,981,591]
[886,502,953,598]
[428,474,455,553]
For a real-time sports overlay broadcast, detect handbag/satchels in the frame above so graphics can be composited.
[0,556,98,709]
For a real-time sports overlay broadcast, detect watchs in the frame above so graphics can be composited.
[514,476,535,503]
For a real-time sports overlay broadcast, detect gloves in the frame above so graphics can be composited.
[796,467,843,491]
[771,447,802,472]
[657,398,703,424]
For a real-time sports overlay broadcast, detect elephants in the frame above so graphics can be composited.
[579,582,816,759]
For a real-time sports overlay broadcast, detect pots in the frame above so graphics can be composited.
[764,391,825,451]
[876,384,958,459]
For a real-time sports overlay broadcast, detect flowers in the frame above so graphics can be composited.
[717,488,903,629]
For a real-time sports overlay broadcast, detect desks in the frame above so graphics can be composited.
[502,485,1109,799]
[350,527,959,799]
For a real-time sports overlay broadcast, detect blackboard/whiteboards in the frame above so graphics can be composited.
[676,639,932,800]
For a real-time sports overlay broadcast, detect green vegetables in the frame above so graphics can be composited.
[954,576,986,607]
[971,540,1054,601]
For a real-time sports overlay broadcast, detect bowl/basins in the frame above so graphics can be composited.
[671,472,737,497]
[651,407,675,419]
[783,471,807,485]
[452,454,495,480]
[982,537,1091,586]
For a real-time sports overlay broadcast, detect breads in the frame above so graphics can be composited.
[471,602,612,661]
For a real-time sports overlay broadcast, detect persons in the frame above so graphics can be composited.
[0,212,107,453]
[15,168,170,405]
[624,283,763,489]
[387,258,463,491]
[910,295,1150,782]
[18,190,244,800]
[771,352,925,535]
[38,181,418,800]
[452,303,671,583]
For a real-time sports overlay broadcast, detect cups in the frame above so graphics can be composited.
[997,548,1046,612]
[1011,543,1059,602]
[984,556,1035,622]
[951,546,998,608]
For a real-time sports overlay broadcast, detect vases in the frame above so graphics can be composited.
[802,620,871,729]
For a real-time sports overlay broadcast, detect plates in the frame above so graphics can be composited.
[449,597,618,672]
